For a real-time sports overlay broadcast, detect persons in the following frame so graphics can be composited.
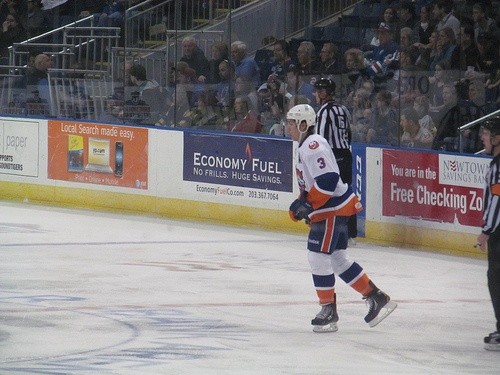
[312,79,357,247]
[12,54,56,117]
[0,0,240,80]
[342,0,500,155]
[109,35,375,139]
[286,103,397,333]
[477,115,500,351]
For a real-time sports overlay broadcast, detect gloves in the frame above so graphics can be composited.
[289,191,313,225]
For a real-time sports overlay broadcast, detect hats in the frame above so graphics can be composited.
[79,11,90,18]
[377,23,392,32]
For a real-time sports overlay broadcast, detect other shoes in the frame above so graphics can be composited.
[347,238,356,245]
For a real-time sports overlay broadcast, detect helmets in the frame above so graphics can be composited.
[286,104,316,127]
[315,78,335,96]
[481,115,500,135]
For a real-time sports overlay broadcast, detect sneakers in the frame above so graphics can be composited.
[362,280,397,327]
[312,293,339,332]
[483,330,500,351]
